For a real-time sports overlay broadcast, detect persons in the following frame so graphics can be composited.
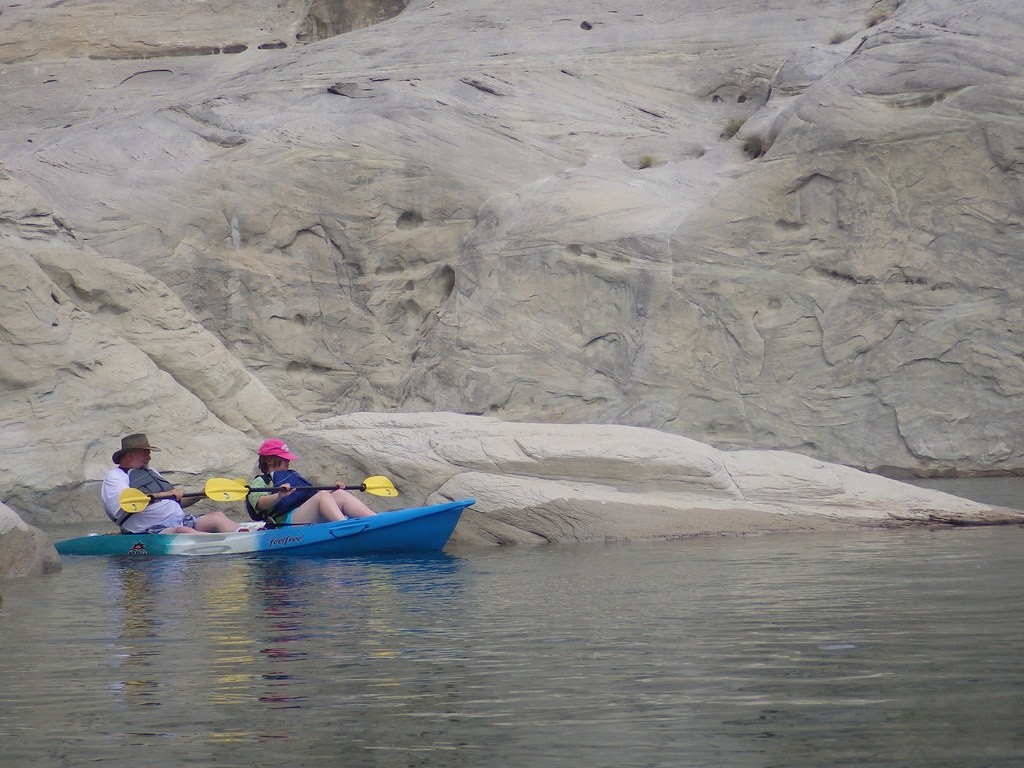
[101,433,250,534]
[245,439,378,529]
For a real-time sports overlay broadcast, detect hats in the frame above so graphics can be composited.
[112,434,161,464]
[258,438,299,460]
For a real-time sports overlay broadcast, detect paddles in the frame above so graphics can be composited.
[118,478,247,513]
[204,475,399,503]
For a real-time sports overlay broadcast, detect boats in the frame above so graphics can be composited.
[53,496,475,559]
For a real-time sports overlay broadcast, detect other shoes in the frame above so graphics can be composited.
[234,527,251,532]
